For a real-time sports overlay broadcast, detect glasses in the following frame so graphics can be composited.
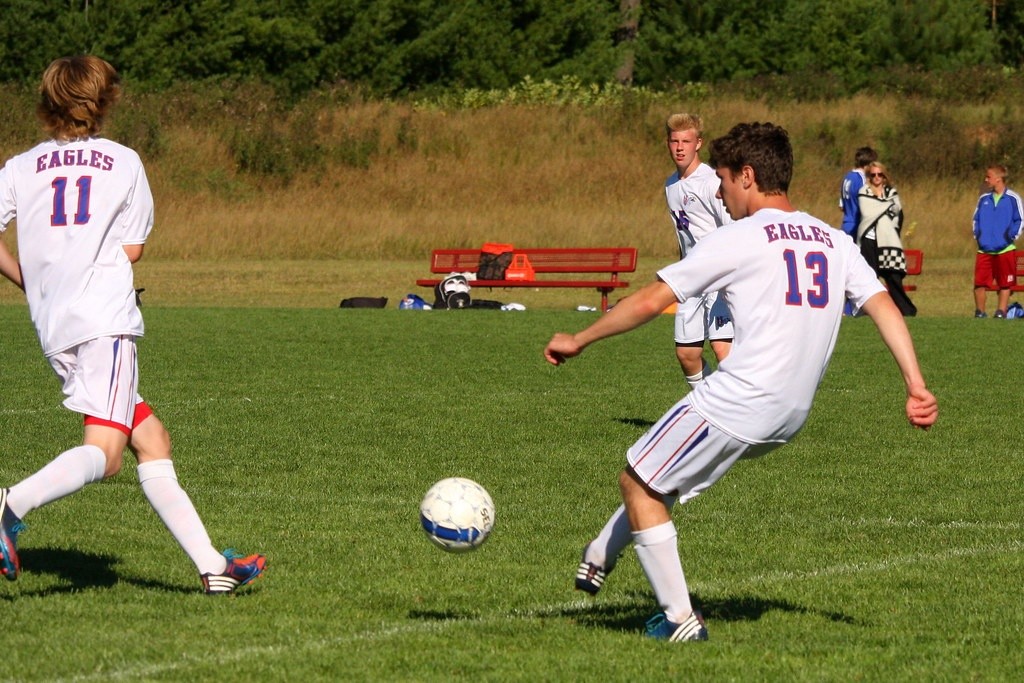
[870,173,884,178]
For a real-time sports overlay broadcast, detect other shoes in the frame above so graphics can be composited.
[994,310,1007,319]
[974,309,986,318]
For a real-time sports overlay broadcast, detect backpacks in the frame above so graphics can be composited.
[432,275,471,310]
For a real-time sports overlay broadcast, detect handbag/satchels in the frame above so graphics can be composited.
[477,242,514,281]
[399,293,432,310]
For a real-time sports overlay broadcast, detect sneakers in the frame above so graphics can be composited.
[577,537,623,596]
[0,487,26,582]
[199,549,267,595]
[620,608,708,644]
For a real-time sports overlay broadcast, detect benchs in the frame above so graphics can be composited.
[416,247,638,313]
[885,249,923,292]
[985,250,1024,292]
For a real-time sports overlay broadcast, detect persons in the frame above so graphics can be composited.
[970,163,1024,320]
[662,108,735,392]
[856,160,919,318]
[0,49,268,599]
[541,117,939,643]
[838,145,879,317]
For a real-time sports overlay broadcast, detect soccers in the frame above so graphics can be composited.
[419,477,495,553]
